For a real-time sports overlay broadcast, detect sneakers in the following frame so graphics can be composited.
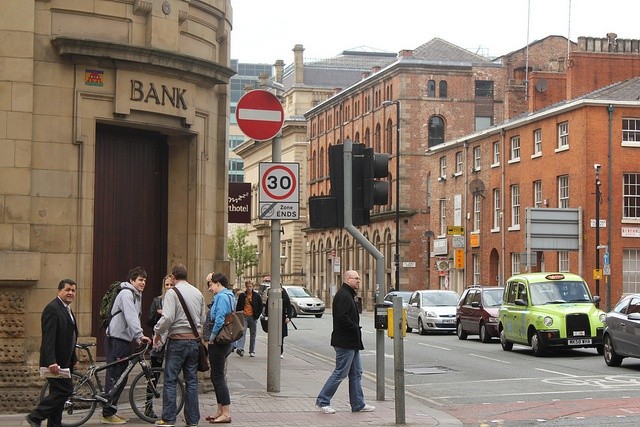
[185,423,198,427]
[355,404,376,412]
[236,348,244,357]
[101,415,126,425]
[154,419,175,427]
[250,352,255,357]
[25,415,40,427]
[281,354,284,359]
[114,413,130,422]
[315,404,337,415]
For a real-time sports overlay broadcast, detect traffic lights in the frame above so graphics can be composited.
[352,142,389,225]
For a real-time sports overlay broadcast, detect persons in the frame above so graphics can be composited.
[152,263,207,427]
[264,282,293,359]
[143,272,177,419]
[387,285,394,294]
[203,271,237,424]
[468,290,481,306]
[230,287,244,352]
[235,279,264,357]
[100,266,152,425]
[24,278,80,427]
[314,269,376,414]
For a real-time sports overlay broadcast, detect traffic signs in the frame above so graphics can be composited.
[258,161,300,220]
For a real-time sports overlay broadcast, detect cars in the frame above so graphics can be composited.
[497,271,606,355]
[455,284,505,343]
[404,288,461,335]
[602,292,639,365]
[383,290,413,309]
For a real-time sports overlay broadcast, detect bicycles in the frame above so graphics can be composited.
[37,335,186,425]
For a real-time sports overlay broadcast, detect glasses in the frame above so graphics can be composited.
[349,277,361,281]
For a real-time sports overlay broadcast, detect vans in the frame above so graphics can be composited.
[260,284,326,317]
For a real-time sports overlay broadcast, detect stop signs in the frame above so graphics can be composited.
[235,89,284,140]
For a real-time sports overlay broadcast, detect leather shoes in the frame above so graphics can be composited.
[206,416,217,421]
[209,417,231,424]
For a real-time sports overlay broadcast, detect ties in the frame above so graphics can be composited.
[66,305,72,319]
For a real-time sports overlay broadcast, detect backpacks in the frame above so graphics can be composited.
[98,281,136,330]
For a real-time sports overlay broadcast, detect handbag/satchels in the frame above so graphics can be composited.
[207,311,245,346]
[260,317,268,333]
[197,344,210,372]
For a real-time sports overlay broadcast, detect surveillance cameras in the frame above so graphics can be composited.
[594,163,606,173]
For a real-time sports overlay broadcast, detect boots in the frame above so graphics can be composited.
[144,402,158,419]
